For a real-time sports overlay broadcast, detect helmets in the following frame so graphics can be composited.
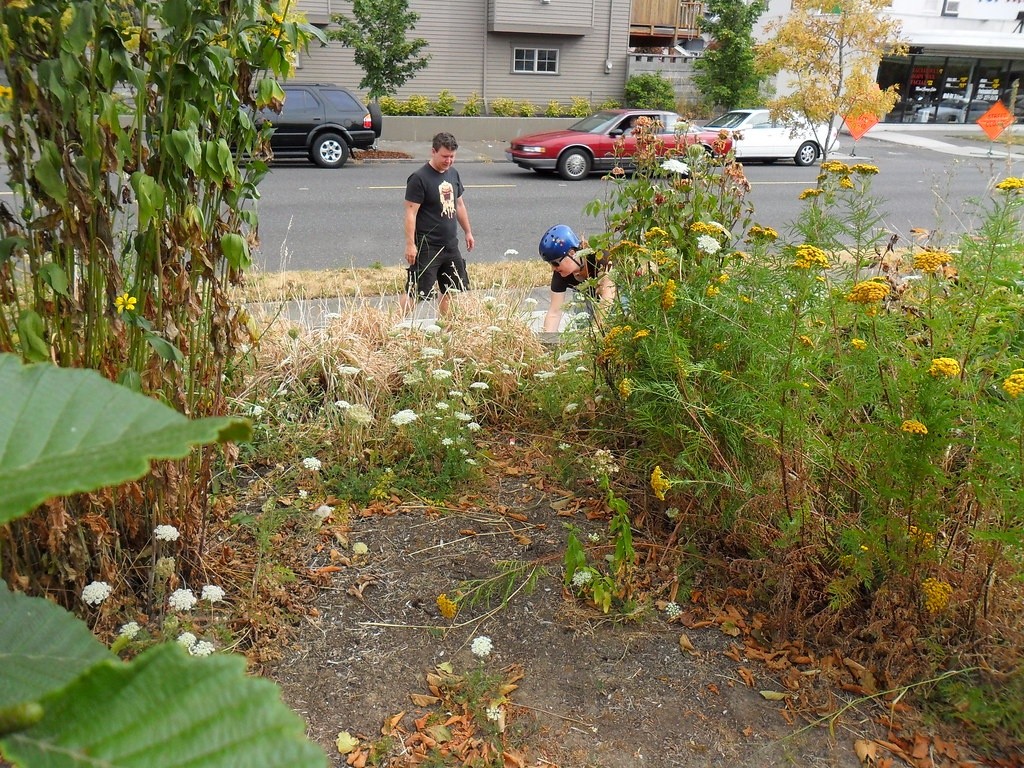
[539,224,583,261]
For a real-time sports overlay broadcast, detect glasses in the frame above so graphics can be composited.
[550,255,566,266]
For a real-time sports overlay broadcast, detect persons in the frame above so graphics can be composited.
[622,117,638,136]
[396,132,475,319]
[539,224,635,333]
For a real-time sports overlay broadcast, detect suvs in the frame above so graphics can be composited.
[226,79,382,169]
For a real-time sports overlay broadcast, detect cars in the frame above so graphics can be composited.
[917,98,996,124]
[703,109,840,166]
[503,107,732,180]
